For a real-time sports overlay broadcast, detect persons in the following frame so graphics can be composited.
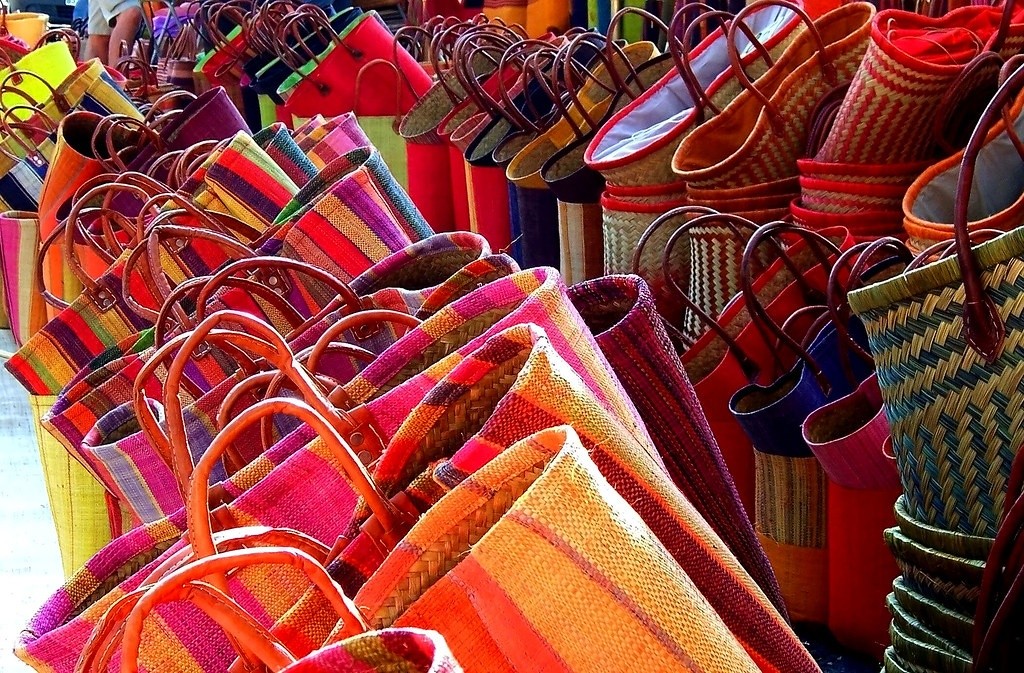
[73,0,143,79]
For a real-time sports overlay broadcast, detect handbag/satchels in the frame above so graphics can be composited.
[0,0,1024,673]
